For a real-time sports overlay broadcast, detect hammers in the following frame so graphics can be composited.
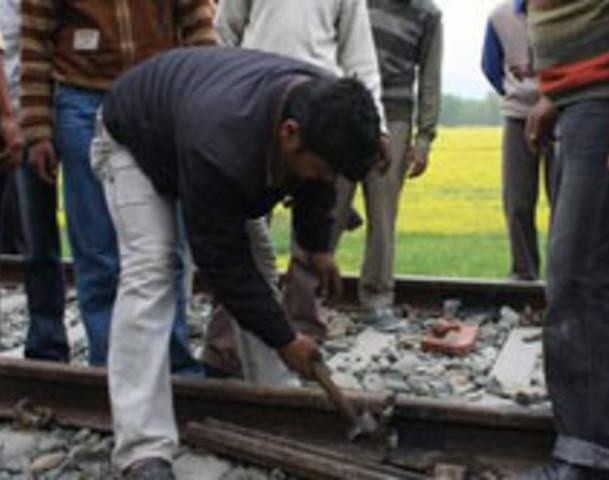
[310,364,378,441]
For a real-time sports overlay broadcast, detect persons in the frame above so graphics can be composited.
[506,0,608,480]
[320,0,443,327]
[210,1,388,364]
[1,1,71,369]
[20,1,217,379]
[481,0,565,283]
[86,43,385,480]
